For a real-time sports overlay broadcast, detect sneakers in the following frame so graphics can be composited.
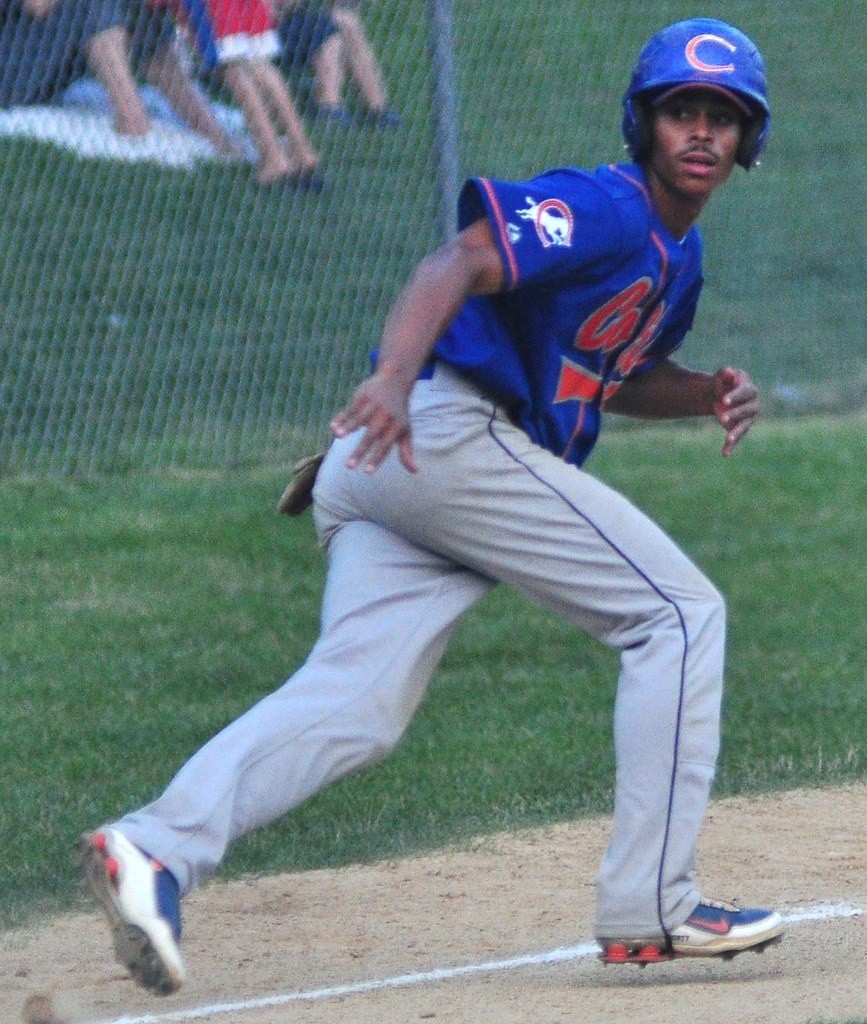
[77,824,183,998]
[596,896,783,964]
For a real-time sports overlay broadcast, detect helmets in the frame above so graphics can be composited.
[621,18,770,172]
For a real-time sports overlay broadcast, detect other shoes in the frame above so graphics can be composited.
[367,111,403,128]
[253,181,292,201]
[316,106,353,123]
[293,168,328,196]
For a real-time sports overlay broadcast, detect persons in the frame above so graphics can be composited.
[70,18,788,995]
[0,0,402,191]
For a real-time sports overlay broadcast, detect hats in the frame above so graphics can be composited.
[647,81,753,117]
